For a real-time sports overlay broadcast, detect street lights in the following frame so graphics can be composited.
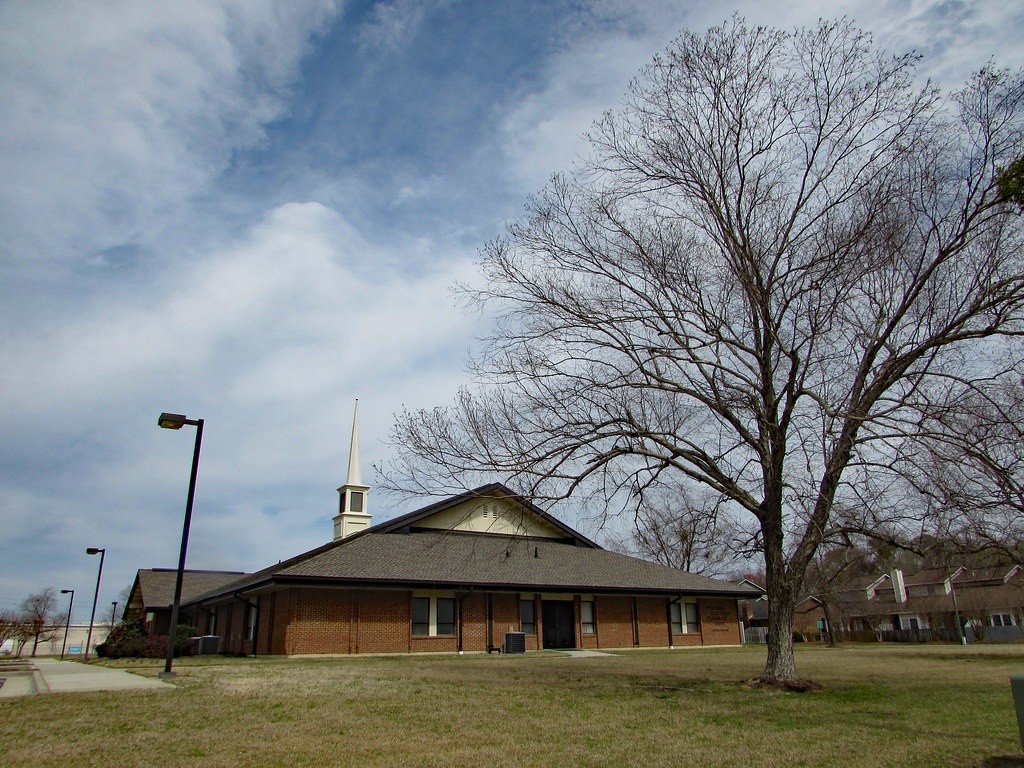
[156,412,205,674]
[84,547,106,657]
[111,601,118,628]
[60,589,76,659]
